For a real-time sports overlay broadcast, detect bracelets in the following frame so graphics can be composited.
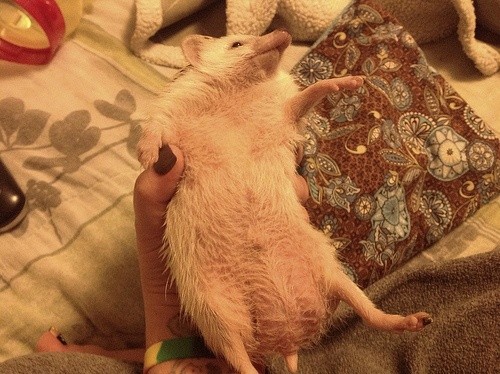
[140,336,218,374]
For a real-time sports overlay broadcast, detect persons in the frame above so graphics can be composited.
[32,141,310,374]
